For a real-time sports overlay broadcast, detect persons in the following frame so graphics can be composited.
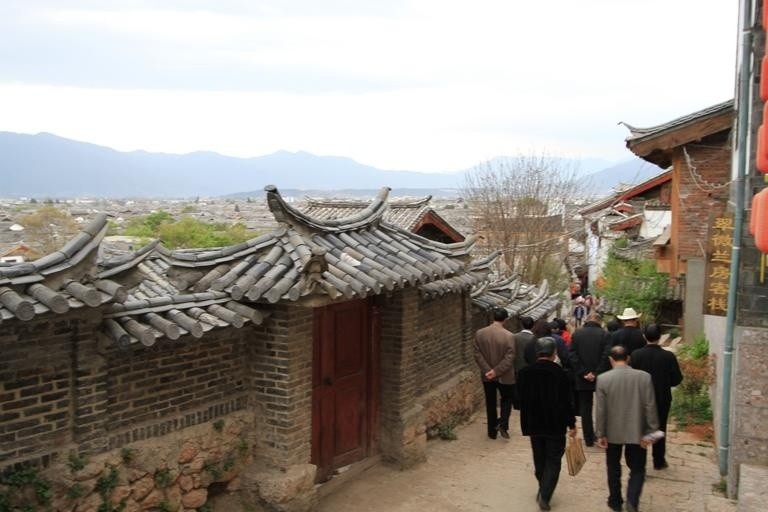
[570,276,593,328]
[472,308,516,440]
[511,308,652,448]
[630,322,682,470]
[594,345,660,512]
[515,336,576,512]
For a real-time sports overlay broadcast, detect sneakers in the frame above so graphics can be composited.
[626,500,641,512]
[537,491,551,511]
[606,496,625,512]
[495,421,509,439]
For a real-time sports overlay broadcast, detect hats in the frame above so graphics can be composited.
[615,308,643,321]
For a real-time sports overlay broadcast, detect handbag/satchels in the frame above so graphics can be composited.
[564,434,587,477]
